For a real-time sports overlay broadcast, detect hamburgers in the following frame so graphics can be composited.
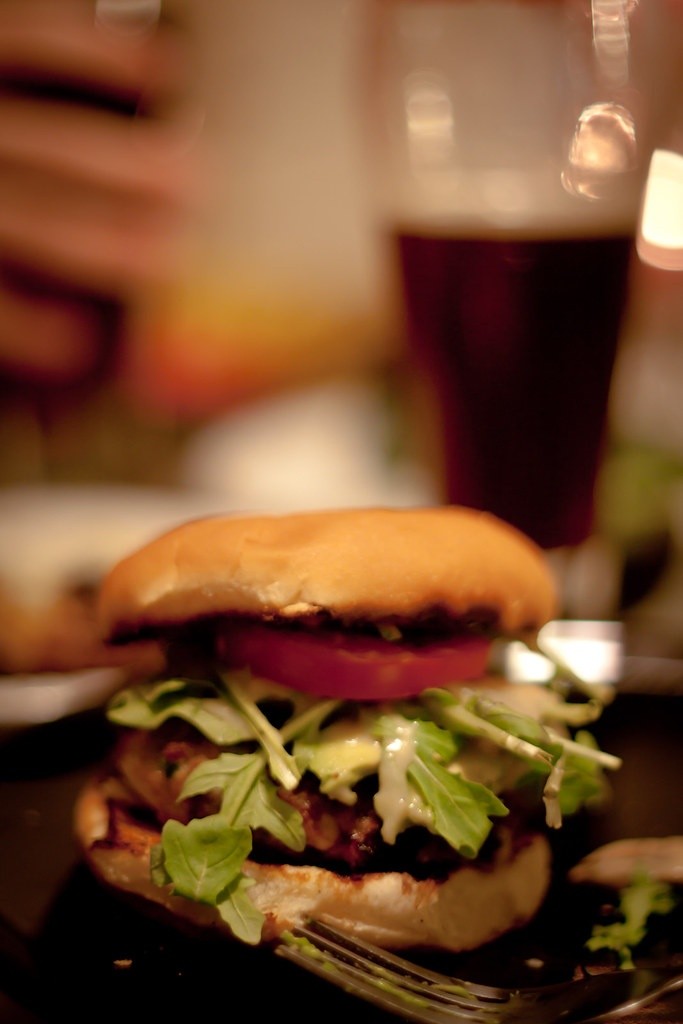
[70,504,624,947]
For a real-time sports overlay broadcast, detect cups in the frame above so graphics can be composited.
[359,1,666,610]
